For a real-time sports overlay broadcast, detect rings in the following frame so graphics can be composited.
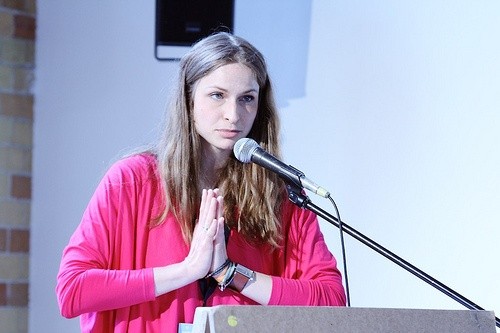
[202,227,208,231]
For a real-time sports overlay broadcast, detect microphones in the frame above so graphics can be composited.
[233,138,330,198]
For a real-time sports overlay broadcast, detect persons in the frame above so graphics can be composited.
[55,33,345,333]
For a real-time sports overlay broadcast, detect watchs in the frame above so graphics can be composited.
[227,263,254,293]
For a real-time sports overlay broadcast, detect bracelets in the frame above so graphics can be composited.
[205,259,241,291]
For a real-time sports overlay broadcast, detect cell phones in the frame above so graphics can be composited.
[154,0,234,61]
